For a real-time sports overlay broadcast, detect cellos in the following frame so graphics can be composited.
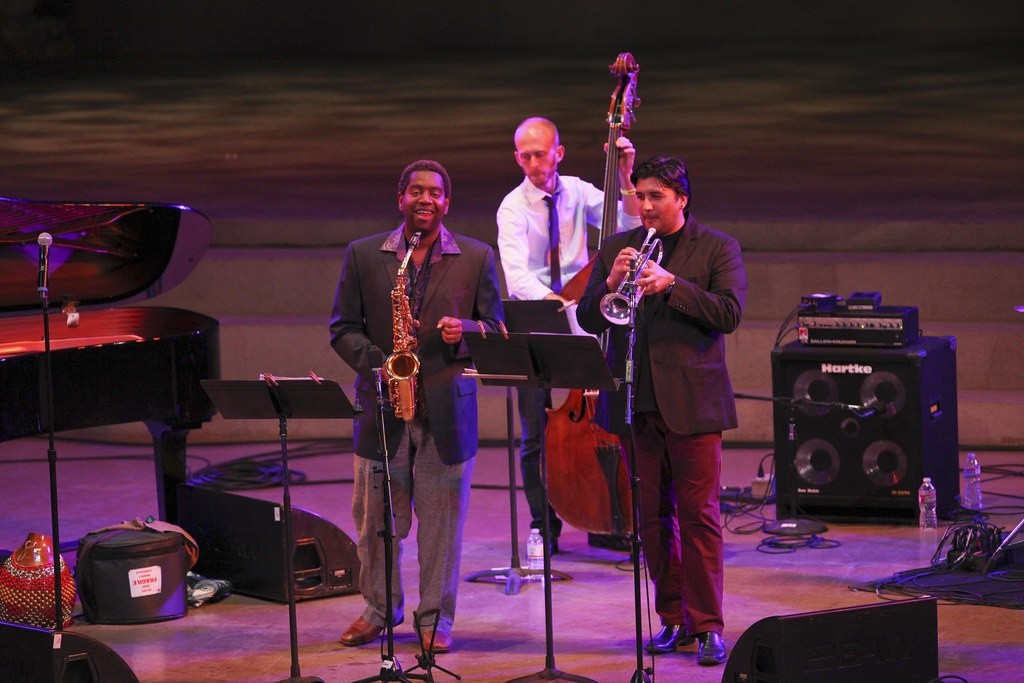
[537,52,641,564]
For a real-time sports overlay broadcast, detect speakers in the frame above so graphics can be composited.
[771,335,960,524]
[720,596,938,683]
[176,482,360,602]
[0,619,140,683]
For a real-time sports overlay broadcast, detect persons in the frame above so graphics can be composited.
[497,117,641,560]
[329,158,502,652]
[576,156,745,666]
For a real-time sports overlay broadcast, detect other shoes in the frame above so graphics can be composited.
[549,533,559,554]
[588,533,632,550]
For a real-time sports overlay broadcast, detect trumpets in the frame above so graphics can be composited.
[600,228,664,325]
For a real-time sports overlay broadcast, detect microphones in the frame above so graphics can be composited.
[369,350,384,409]
[862,401,887,417]
[37,232,53,298]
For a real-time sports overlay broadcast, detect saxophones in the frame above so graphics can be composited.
[381,231,422,424]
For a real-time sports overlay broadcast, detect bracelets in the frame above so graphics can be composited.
[606,276,613,292]
[619,187,635,196]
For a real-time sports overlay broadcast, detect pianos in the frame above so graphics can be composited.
[0,196,224,536]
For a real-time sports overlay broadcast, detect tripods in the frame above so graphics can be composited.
[348,299,618,683]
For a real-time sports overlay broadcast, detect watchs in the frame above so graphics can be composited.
[664,281,675,294]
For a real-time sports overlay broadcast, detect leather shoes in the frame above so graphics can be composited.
[339,616,404,644]
[646,624,695,655]
[697,631,726,664]
[421,630,453,652]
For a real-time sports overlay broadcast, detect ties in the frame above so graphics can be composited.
[543,191,562,293]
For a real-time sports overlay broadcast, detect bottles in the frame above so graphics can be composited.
[918,477,936,534]
[963,453,981,509]
[527,528,544,581]
